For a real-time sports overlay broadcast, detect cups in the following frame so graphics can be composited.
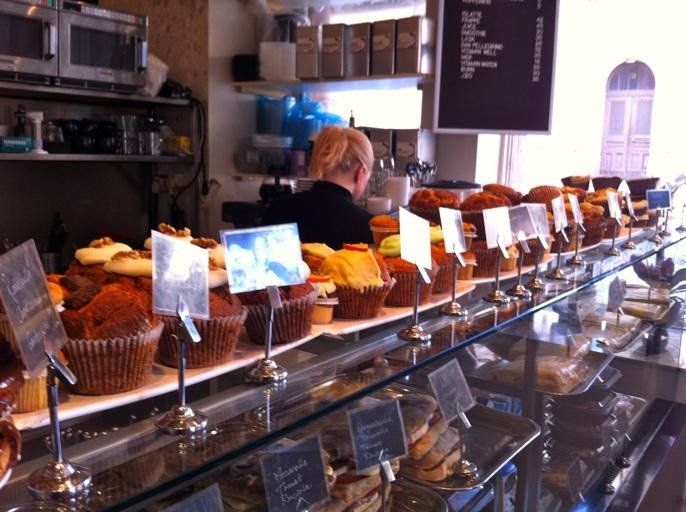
[5,363,54,419]
[60,319,162,398]
[2,102,173,161]
[244,289,323,347]
[140,303,250,371]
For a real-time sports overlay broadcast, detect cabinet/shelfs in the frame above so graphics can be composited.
[0,80,193,274]
[0,230,686,512]
[231,70,433,214]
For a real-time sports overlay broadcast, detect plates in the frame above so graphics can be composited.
[306,170,662,326]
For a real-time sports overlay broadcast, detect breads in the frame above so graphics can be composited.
[97,376,466,510]
[496,354,587,394]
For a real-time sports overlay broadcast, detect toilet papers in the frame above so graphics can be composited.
[259,41,296,79]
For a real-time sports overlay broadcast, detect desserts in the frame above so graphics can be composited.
[1,176,662,415]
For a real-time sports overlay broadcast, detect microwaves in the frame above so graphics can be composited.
[57,0,150,95]
[1,0,56,84]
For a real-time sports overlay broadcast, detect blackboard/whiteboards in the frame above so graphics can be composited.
[431,0,560,135]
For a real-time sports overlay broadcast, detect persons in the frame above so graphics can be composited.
[258,125,379,251]
